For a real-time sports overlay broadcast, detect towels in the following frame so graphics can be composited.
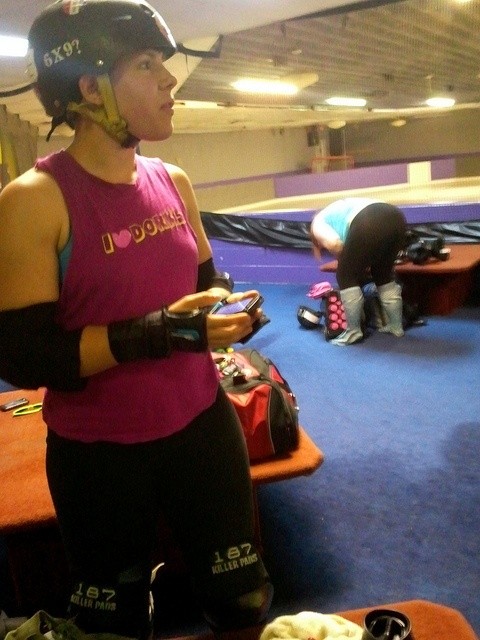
[260,609,364,640]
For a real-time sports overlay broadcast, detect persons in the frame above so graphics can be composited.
[0,1,277,638]
[310,197,407,347]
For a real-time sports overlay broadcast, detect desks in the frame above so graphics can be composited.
[0,385,323,562]
[333,599,478,639]
[318,246,479,317]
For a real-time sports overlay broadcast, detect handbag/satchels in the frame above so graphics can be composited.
[323,288,347,340]
[211,350,298,462]
[362,281,418,330]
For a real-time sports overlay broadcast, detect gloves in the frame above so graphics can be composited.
[109,305,206,365]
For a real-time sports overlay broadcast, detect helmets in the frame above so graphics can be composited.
[28,0,176,115]
[297,305,321,328]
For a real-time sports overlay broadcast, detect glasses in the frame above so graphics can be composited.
[13,402,43,415]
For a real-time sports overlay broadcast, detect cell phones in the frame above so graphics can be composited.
[207,295,264,313]
[0,398,29,412]
